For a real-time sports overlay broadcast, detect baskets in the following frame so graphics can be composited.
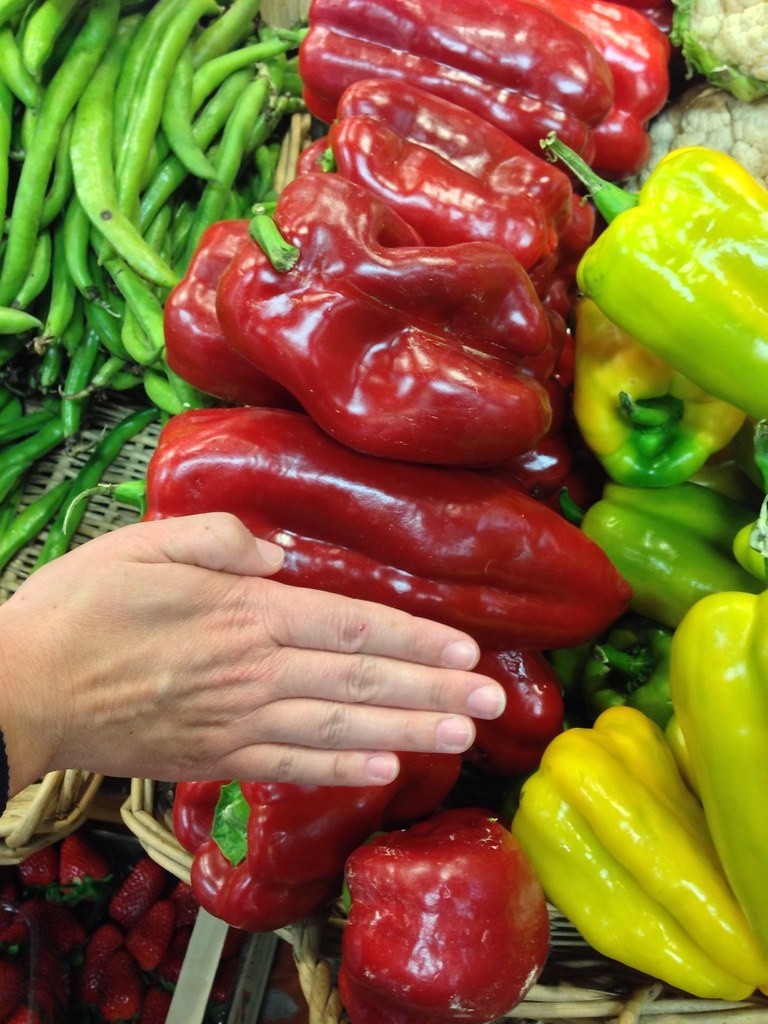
[0,345,768,1022]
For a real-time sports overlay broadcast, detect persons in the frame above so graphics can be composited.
[0,512,511,824]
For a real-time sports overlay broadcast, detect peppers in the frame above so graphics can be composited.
[0,0,768,1022]
